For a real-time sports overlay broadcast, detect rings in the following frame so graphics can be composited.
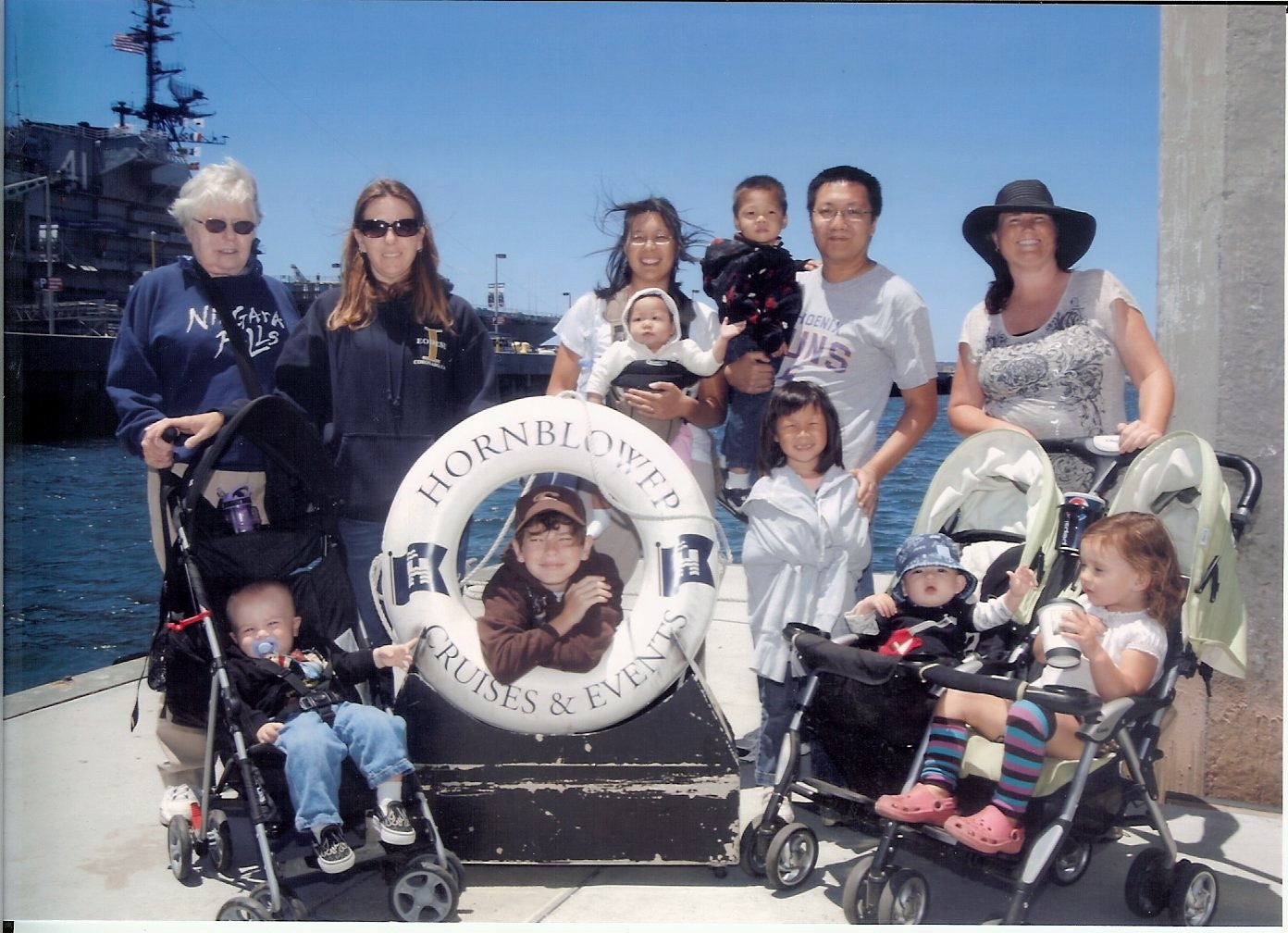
[650,406,653,412]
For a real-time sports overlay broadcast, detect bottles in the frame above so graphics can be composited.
[217,483,254,534]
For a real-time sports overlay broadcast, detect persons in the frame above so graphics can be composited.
[218,574,418,874]
[740,381,872,828]
[731,164,938,764]
[276,177,500,709]
[476,485,624,685]
[585,288,745,406]
[947,179,1174,496]
[546,196,727,516]
[702,175,822,523]
[846,532,1038,680]
[106,160,299,826]
[874,511,1185,856]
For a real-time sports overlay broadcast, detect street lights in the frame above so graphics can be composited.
[494,254,506,351]
[563,292,571,308]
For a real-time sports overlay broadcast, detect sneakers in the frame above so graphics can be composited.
[717,479,752,523]
[160,783,202,825]
[759,784,794,825]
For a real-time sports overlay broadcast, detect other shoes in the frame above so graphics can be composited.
[371,800,416,846]
[311,823,356,874]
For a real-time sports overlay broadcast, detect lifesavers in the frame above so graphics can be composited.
[381,396,719,735]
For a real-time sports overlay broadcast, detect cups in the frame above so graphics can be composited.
[1036,597,1087,669]
[1056,491,1106,554]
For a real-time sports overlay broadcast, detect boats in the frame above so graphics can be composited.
[1,0,233,440]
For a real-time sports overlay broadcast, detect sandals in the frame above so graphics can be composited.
[944,805,1026,854]
[874,781,963,829]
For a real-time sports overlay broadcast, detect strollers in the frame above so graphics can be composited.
[736,426,1265,926]
[128,388,470,921]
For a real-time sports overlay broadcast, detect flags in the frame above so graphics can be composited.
[113,32,144,55]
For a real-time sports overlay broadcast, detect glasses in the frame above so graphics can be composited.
[192,217,257,234]
[358,218,423,238]
[809,207,874,219]
[626,234,678,245]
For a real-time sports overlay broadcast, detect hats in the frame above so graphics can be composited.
[891,533,978,607]
[962,179,1097,270]
[514,485,586,531]
